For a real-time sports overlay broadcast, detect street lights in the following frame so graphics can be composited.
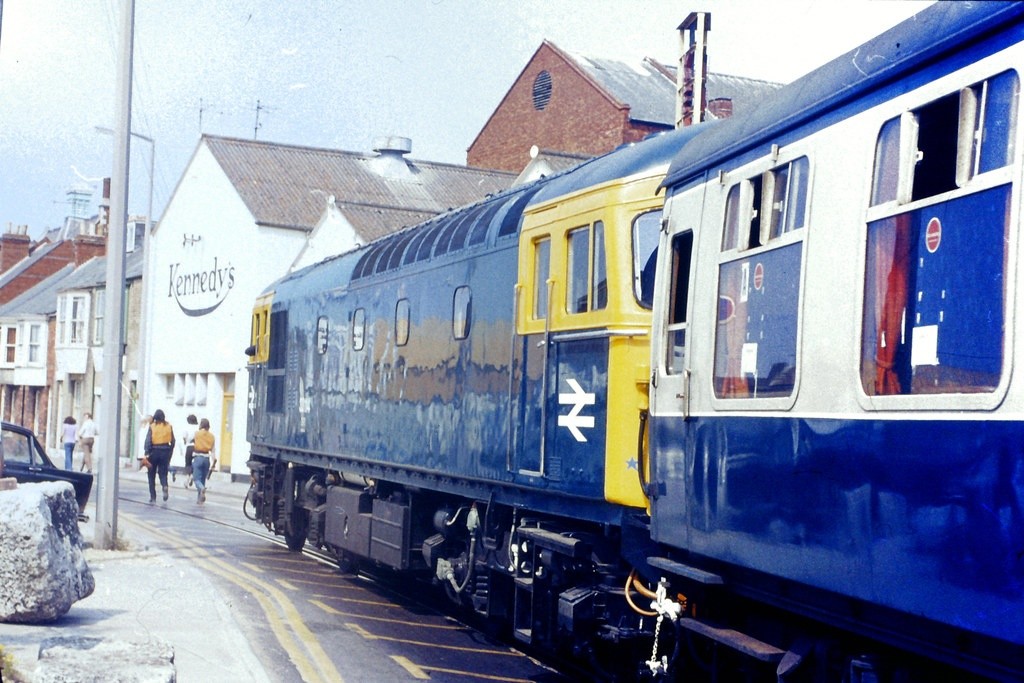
[93,122,156,469]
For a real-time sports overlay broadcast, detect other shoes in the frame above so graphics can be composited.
[197,501,203,504]
[149,496,156,502]
[189,474,194,486]
[200,487,206,502]
[163,485,168,501]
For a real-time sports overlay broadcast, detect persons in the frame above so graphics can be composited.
[59,413,98,473]
[136,409,216,504]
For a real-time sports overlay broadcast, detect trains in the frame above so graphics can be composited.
[243,0,1024,682]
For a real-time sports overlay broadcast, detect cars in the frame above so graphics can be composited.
[1,422,96,522]
